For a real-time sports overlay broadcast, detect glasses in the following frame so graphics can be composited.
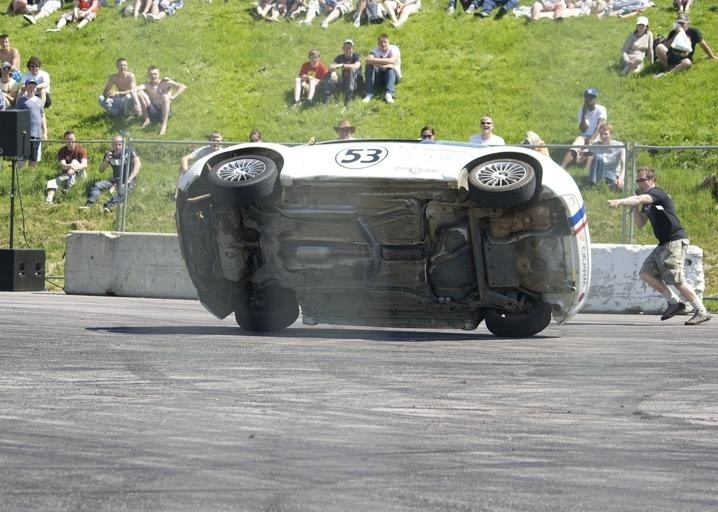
[423,135,432,139]
[481,122,492,125]
[636,175,651,183]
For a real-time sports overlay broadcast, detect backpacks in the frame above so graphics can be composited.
[654,34,666,60]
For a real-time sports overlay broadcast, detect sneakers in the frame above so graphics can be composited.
[684,312,711,324]
[661,301,685,320]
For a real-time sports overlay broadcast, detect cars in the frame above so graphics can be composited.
[173,139,594,344]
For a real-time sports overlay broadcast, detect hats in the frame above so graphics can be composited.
[345,40,353,46]
[1,62,11,68]
[25,78,38,85]
[636,16,649,26]
[334,120,356,133]
[676,15,688,23]
[584,88,596,96]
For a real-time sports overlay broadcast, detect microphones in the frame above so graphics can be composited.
[629,188,642,214]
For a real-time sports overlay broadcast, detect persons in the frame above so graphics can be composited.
[14,77,48,168]
[559,89,609,172]
[653,15,718,79]
[46,131,88,204]
[323,40,362,110]
[114,0,185,22]
[520,130,549,157]
[0,34,51,111]
[470,117,505,145]
[362,34,403,103]
[583,123,627,192]
[107,66,187,136]
[181,130,223,171]
[79,135,141,212]
[251,0,422,29]
[421,126,436,140]
[334,120,358,139]
[99,58,142,123]
[250,130,262,142]
[1,1,99,32]
[292,49,328,107]
[619,16,655,76]
[448,1,693,21]
[606,166,712,325]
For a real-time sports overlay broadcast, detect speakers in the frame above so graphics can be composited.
[0,108,31,161]
[0,248,46,292]
